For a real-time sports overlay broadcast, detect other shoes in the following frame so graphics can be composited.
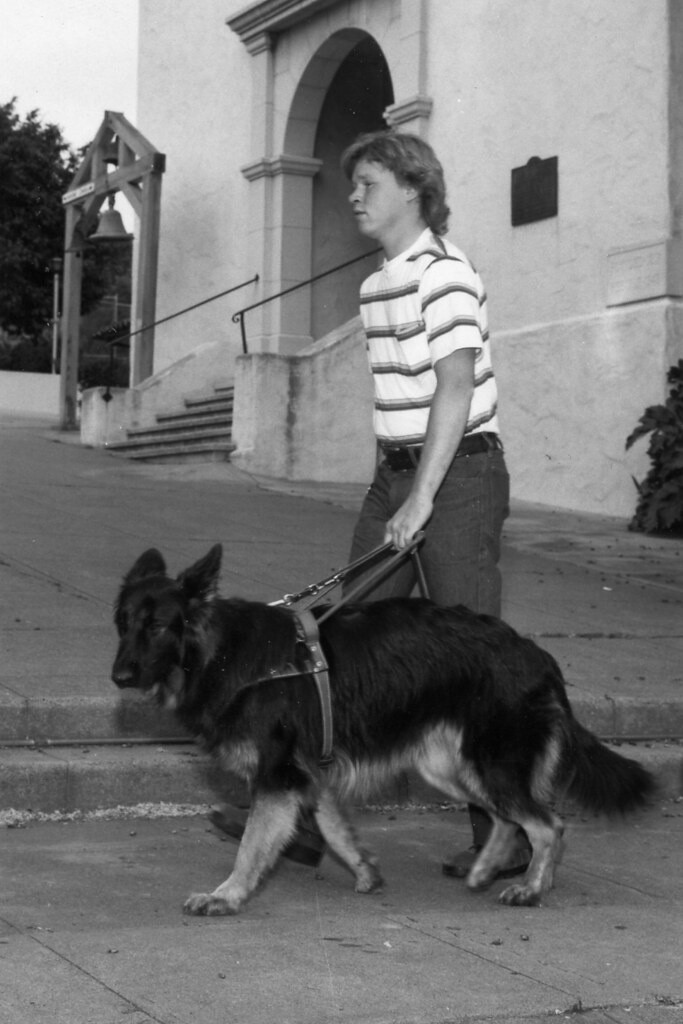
[206,802,327,869]
[442,844,532,878]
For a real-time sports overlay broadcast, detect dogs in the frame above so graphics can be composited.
[110,543,651,914]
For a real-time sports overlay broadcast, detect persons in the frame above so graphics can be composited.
[208,133,535,879]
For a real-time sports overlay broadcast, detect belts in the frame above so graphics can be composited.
[381,432,499,471]
